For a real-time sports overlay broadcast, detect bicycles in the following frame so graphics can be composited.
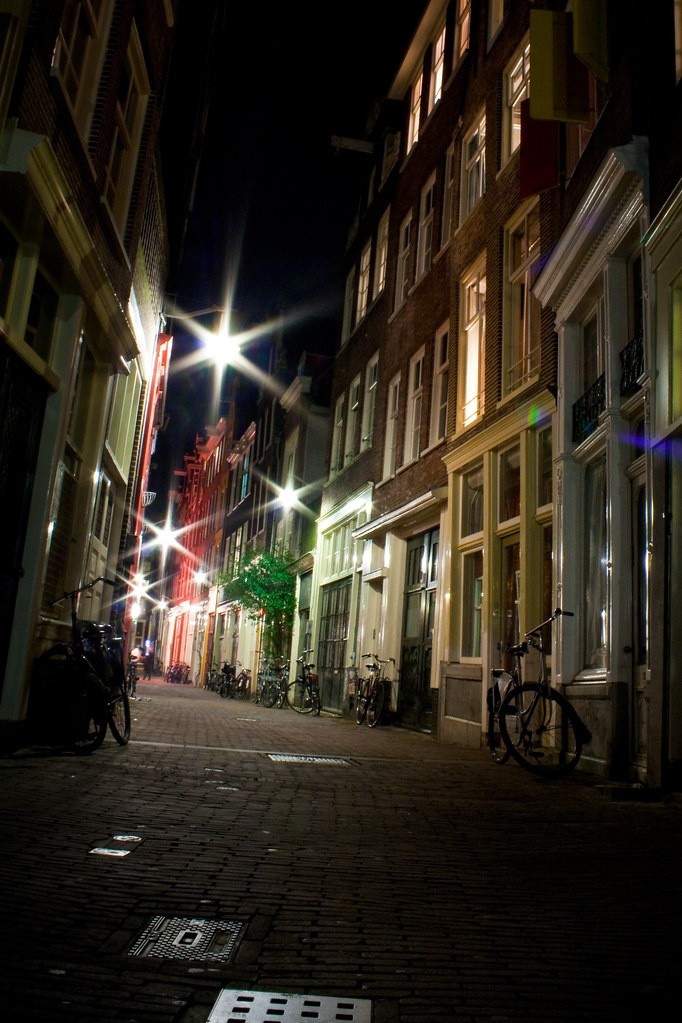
[204,659,252,701]
[254,650,293,710]
[355,652,396,729]
[163,658,191,684]
[27,576,132,756]
[286,648,323,717]
[123,654,140,698]
[486,608,593,777]
